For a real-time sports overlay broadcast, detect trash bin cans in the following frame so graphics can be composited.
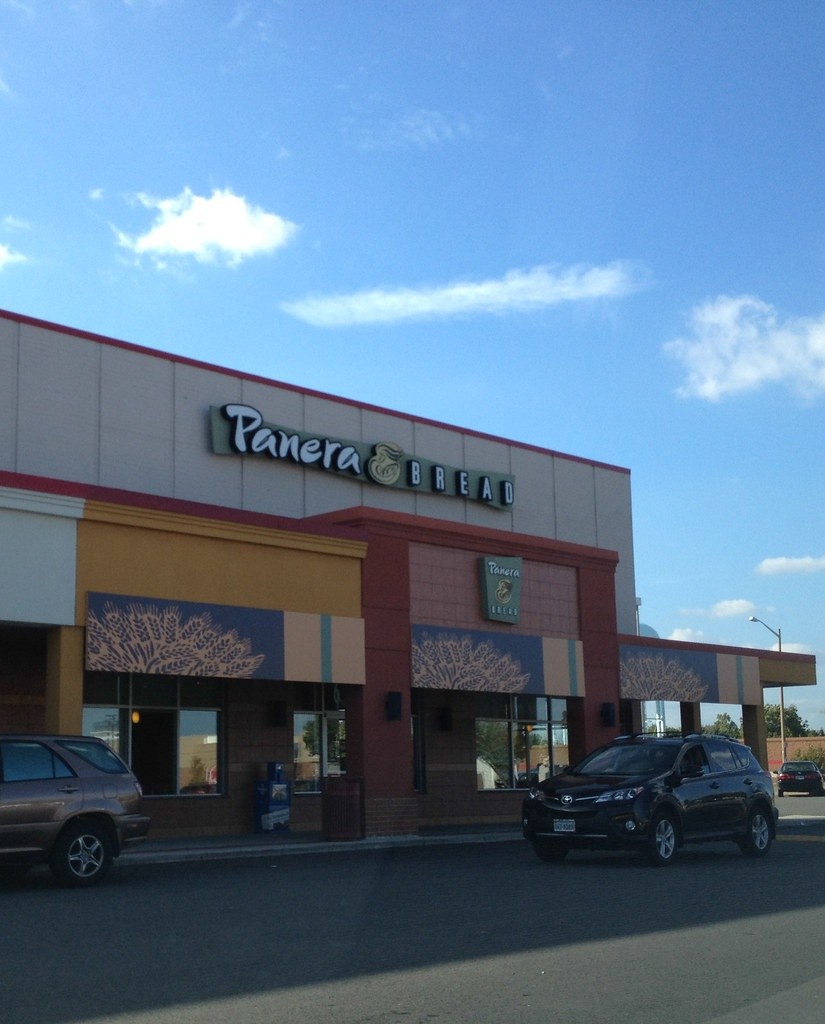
[318,776,363,841]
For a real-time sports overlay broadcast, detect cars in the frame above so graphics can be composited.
[772,761,825,797]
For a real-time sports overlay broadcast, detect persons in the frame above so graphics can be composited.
[648,750,695,779]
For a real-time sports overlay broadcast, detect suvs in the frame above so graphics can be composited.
[521,729,781,867]
[0,734,151,890]
[494,760,569,790]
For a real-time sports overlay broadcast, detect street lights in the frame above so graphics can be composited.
[747,616,788,764]
